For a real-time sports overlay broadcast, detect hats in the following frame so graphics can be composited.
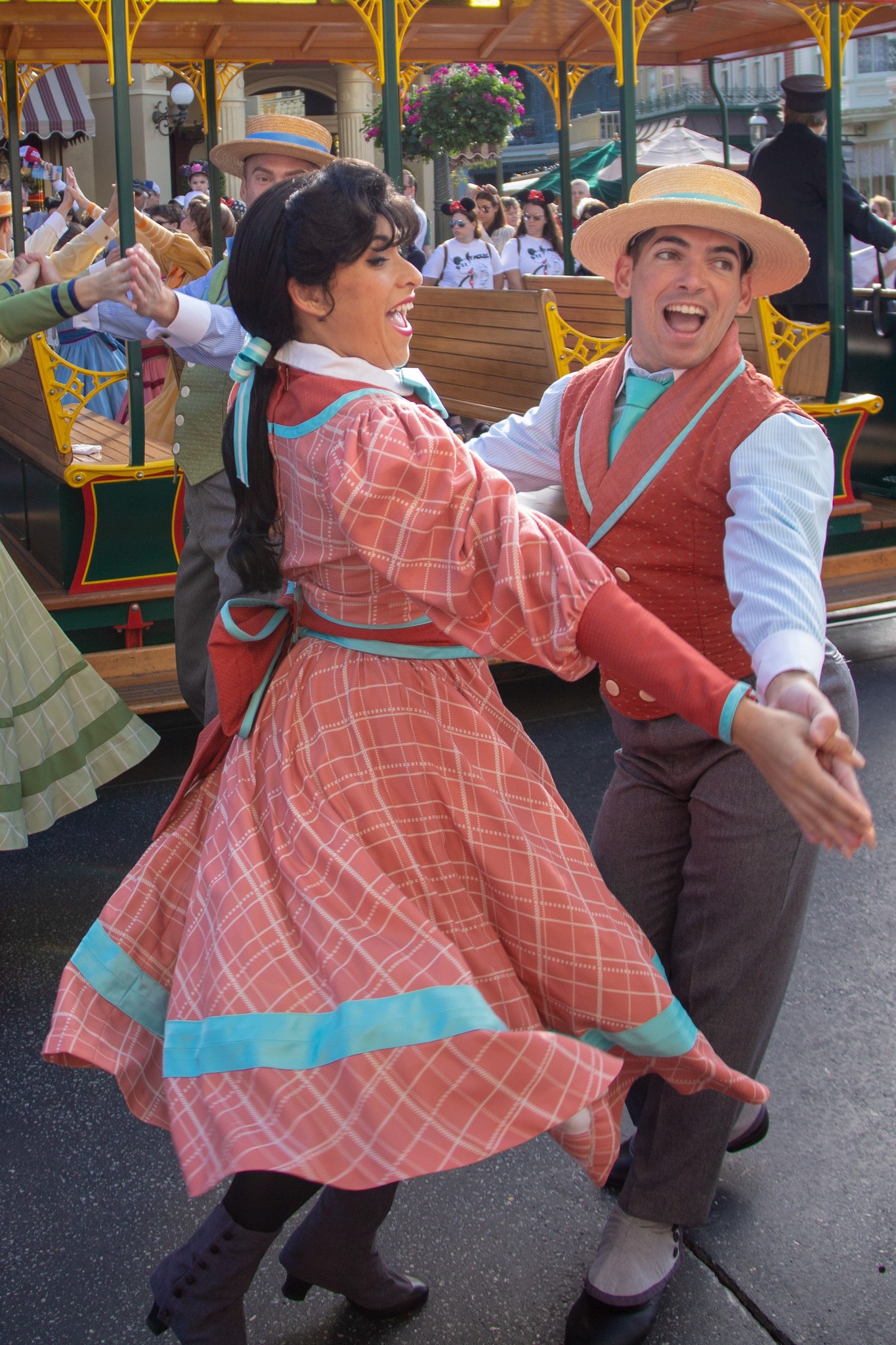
[781,76,830,112]
[19,145,40,163]
[168,196,185,206]
[144,180,161,194]
[209,114,337,178]
[132,178,146,194]
[570,165,811,298]
[0,191,30,217]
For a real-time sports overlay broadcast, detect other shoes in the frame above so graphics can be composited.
[564,1229,683,1345]
[605,1095,769,1196]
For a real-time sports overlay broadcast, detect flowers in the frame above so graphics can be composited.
[361,57,528,162]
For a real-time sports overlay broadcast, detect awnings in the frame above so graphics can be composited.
[0,64,96,151]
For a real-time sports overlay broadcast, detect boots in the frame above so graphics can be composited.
[281,1180,429,1312]
[146,1203,279,1345]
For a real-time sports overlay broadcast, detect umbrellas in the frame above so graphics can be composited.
[597,117,750,181]
[510,132,645,205]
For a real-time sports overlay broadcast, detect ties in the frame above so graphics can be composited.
[606,373,676,468]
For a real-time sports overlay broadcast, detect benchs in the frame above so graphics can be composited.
[0,332,186,595]
[410,286,626,426]
[522,272,885,534]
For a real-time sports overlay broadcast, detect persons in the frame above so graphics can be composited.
[473,182,517,289]
[12,112,335,729]
[571,198,610,276]
[501,188,565,291]
[0,163,249,451]
[0,255,164,850]
[868,195,894,223]
[421,197,505,289]
[397,228,426,273]
[223,165,860,1345]
[501,196,522,233]
[747,74,896,393]
[413,177,432,260]
[41,154,871,1345]
[554,179,591,236]
[401,168,425,249]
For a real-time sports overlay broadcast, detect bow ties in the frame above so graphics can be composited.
[400,366,449,418]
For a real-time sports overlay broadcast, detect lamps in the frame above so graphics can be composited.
[153,82,193,137]
[841,133,868,163]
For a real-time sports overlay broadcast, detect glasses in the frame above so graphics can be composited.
[523,212,546,220]
[147,193,156,199]
[872,209,884,214]
[21,159,35,167]
[581,215,590,221]
[156,220,172,226]
[403,185,412,190]
[449,219,472,227]
[478,204,495,213]
